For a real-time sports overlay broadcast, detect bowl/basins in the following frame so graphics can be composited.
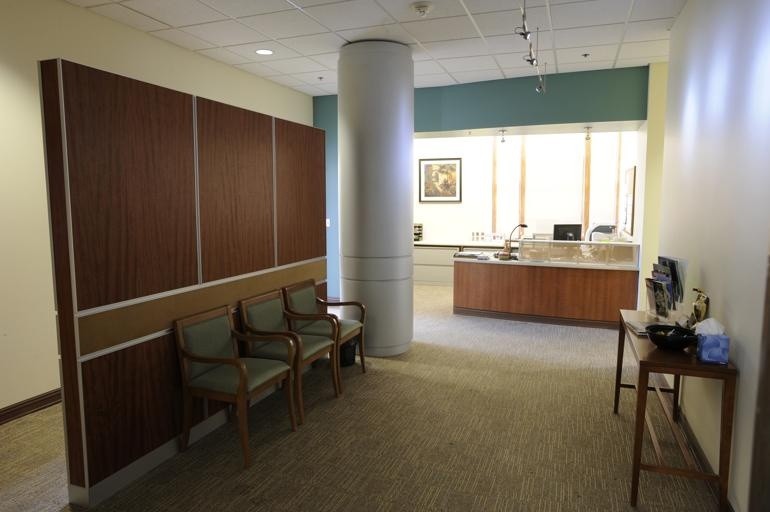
[646,349,693,364]
[645,323,696,349]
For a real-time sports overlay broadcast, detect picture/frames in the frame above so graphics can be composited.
[418,158,463,203]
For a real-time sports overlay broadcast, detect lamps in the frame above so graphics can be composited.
[508,223,528,260]
[514,2,546,96]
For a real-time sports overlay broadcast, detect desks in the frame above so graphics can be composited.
[612,309,739,510]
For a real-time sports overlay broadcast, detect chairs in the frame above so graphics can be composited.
[173,278,369,471]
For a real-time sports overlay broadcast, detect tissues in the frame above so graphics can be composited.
[691,318,730,364]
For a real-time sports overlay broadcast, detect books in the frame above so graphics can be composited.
[657,255,683,303]
[652,270,674,311]
[645,278,669,318]
[625,320,673,337]
[653,263,677,310]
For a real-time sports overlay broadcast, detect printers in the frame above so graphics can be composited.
[588,225,618,242]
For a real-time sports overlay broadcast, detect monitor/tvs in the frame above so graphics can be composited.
[552,224,582,241]
